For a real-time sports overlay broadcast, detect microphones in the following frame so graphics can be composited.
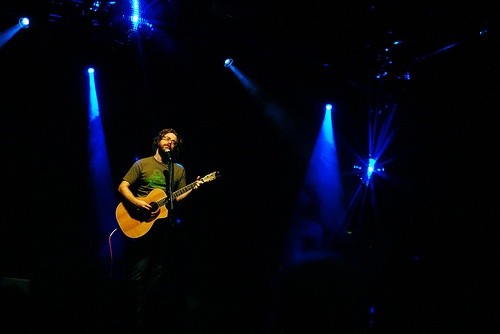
[165,141,172,157]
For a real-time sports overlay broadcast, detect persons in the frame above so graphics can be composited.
[119,130,204,271]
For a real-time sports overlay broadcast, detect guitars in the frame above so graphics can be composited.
[116,171,220,239]
